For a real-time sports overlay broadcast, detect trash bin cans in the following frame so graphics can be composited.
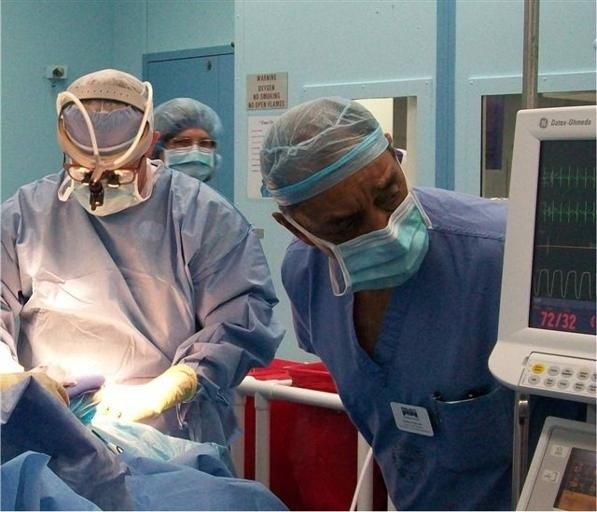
[244,358,388,511]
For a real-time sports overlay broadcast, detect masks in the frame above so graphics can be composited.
[286,157,433,297]
[72,158,151,216]
[161,147,216,182]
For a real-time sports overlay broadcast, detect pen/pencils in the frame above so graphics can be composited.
[431,388,478,402]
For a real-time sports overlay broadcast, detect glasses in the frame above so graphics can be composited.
[171,136,217,149]
[62,153,146,186]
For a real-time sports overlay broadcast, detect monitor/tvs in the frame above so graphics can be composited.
[488,105,596,405]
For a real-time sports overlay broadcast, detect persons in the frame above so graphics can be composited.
[1,64,288,480]
[258,95,590,511]
[148,94,223,184]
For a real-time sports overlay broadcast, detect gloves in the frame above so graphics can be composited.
[2,370,71,407]
[94,364,196,423]
